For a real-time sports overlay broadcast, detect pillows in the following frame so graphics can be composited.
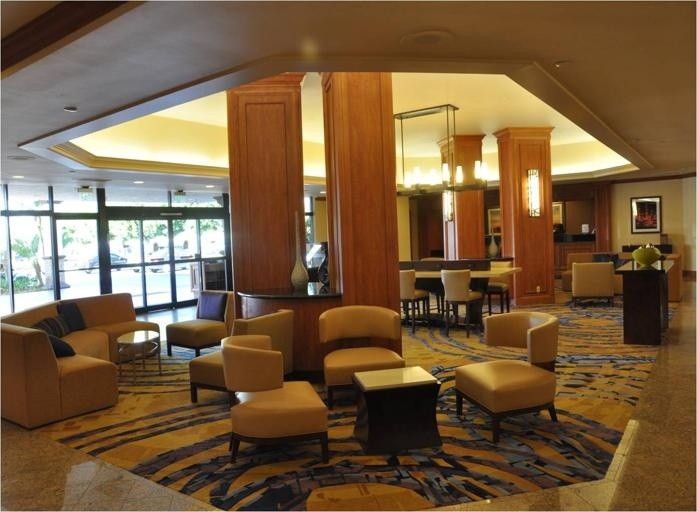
[198,291,227,322]
[617,258,634,269]
[28,316,70,336]
[42,332,76,358]
[57,302,87,333]
[589,252,619,269]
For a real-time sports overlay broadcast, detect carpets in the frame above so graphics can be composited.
[3,298,681,512]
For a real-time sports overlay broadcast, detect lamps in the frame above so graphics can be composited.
[388,101,490,197]
[526,168,542,218]
[441,187,454,224]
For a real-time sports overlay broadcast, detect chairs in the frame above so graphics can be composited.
[393,250,524,337]
[568,262,616,307]
[166,290,235,358]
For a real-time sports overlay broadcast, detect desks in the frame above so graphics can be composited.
[621,242,673,254]
[616,258,677,347]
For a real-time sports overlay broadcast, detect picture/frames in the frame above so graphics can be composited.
[550,201,566,234]
[487,207,503,237]
[629,195,662,234]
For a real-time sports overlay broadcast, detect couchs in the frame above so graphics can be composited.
[559,250,682,307]
[2,292,162,433]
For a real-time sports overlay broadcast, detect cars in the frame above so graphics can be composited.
[84,235,224,274]
